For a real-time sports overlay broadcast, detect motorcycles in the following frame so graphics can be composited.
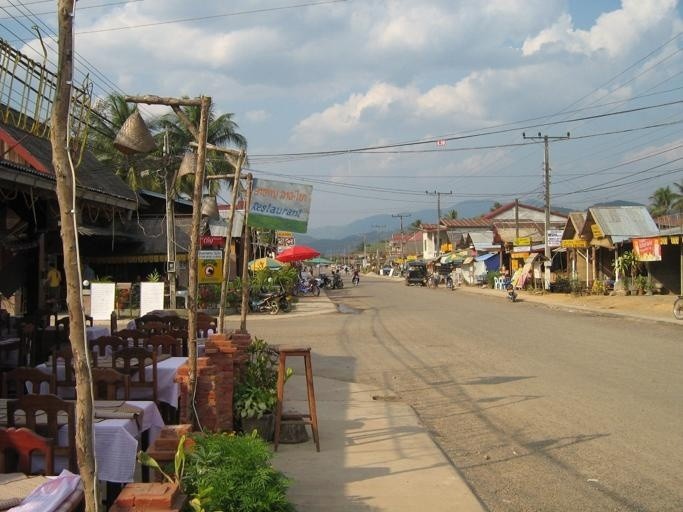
[446,275,454,291]
[351,276,360,287]
[249,273,344,315]
[503,279,517,302]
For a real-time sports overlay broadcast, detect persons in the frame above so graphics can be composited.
[298,264,360,290]
[433,267,451,288]
[45,258,96,315]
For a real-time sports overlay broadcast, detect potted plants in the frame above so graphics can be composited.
[235,336,294,442]
[634,275,654,295]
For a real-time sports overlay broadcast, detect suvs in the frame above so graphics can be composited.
[405,263,427,287]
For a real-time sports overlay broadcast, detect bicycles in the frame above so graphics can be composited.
[672,296,683,319]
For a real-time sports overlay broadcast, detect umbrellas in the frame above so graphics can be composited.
[304,256,334,265]
[248,256,288,273]
[275,246,321,261]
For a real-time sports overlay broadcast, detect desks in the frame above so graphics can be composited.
[0,475,84,512]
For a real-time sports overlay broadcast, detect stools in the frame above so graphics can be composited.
[273,344,321,451]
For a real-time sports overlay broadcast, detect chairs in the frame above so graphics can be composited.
[2,311,217,476]
